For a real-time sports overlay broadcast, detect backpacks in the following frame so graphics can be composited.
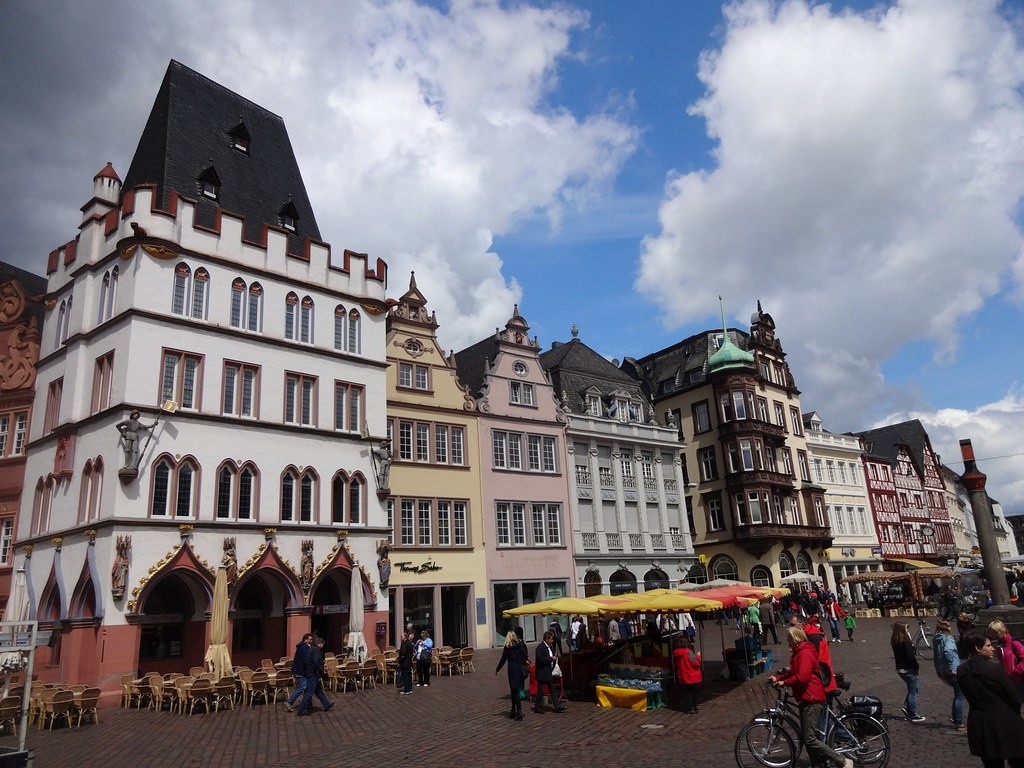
[548,624,560,642]
[811,660,832,686]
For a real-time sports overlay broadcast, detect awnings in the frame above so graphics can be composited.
[885,558,942,588]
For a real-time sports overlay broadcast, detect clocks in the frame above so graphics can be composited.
[922,526,934,536]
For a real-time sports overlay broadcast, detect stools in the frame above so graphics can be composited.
[854,607,937,618]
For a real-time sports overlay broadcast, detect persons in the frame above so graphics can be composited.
[283,633,335,716]
[395,622,434,695]
[1004,569,1024,599]
[988,620,1024,705]
[781,581,855,642]
[889,622,926,722]
[932,619,968,731]
[957,629,1024,768]
[805,623,857,746]
[115,409,158,469]
[760,599,782,645]
[495,610,703,721]
[111,549,129,590]
[371,440,393,490]
[942,585,960,621]
[748,600,763,647]
[713,611,729,625]
[769,625,853,768]
[957,612,976,640]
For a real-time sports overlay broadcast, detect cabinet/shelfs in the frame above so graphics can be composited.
[594,673,674,713]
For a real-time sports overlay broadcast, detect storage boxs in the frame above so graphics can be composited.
[722,648,774,678]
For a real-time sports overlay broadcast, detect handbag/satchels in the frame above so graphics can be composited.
[686,623,696,636]
[566,628,574,646]
[521,665,528,679]
[421,648,431,663]
[551,658,562,677]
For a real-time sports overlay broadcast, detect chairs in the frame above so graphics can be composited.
[118,646,476,716]
[0,680,102,737]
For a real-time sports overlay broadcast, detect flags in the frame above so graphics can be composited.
[360,427,371,439]
[160,399,178,413]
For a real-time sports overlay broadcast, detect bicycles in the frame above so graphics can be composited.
[939,595,977,622]
[889,616,936,661]
[733,680,892,768]
[745,667,890,768]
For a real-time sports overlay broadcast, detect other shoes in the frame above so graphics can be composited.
[901,707,967,730]
[510,711,517,717]
[284,701,293,712]
[555,705,568,713]
[685,709,698,713]
[534,707,546,715]
[399,690,413,695]
[417,684,421,687]
[838,639,841,642]
[832,638,836,643]
[690,638,695,642]
[297,710,311,716]
[514,714,523,720]
[308,705,316,711]
[423,684,428,687]
[325,702,334,710]
[843,759,854,768]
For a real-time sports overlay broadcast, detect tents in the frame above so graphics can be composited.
[1001,554,1024,564]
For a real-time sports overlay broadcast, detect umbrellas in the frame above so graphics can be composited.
[205,563,236,678]
[501,578,791,692]
[780,571,819,592]
[1,565,31,696]
[346,561,368,664]
